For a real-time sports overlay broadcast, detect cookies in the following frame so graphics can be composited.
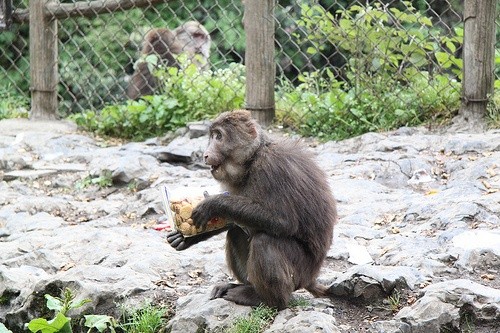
[169,200,227,236]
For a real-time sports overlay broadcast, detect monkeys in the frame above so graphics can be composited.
[166,110,337,311]
[126,21,211,100]
